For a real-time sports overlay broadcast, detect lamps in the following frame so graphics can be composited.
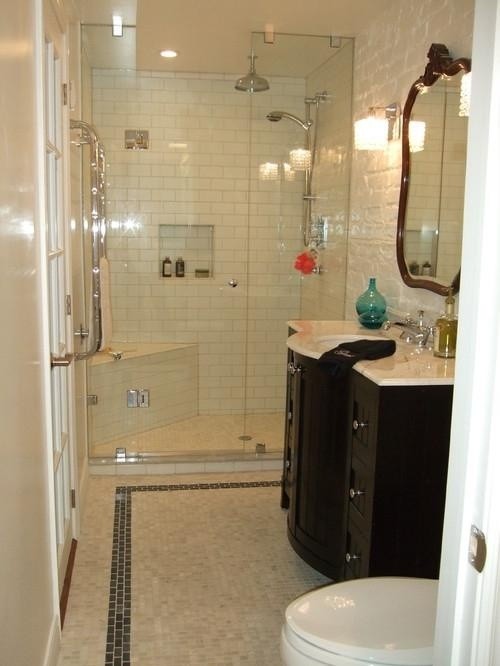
[353,99,401,152]
[458,70,471,118]
[408,118,427,153]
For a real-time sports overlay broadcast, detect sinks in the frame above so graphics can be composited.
[318,336,383,346]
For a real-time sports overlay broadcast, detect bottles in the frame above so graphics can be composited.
[163,255,172,276]
[422,260,432,277]
[356,278,387,320]
[176,256,185,277]
[409,259,420,275]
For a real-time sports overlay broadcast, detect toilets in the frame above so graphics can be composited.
[280,576,439,666]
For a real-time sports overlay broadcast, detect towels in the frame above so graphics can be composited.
[308,338,397,396]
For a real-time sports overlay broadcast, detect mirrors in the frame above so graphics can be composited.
[393,40,472,298]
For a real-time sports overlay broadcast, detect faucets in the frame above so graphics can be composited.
[384,321,428,344]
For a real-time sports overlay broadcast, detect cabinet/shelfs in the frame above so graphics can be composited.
[280,327,453,583]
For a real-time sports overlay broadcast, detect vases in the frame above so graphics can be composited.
[355,278,389,329]
